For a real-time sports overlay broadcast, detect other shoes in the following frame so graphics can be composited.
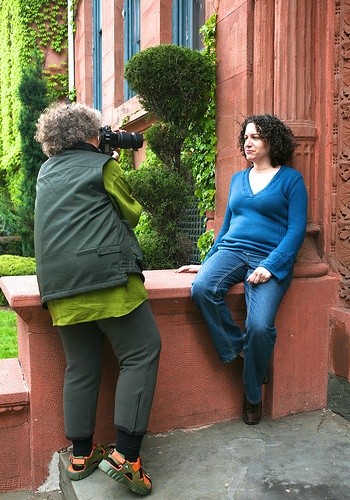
[263,373,270,384]
[243,394,263,425]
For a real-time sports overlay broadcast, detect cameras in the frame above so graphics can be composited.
[97,125,143,157]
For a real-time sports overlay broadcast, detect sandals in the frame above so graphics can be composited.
[67,444,153,497]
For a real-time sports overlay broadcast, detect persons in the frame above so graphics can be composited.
[175,115,309,424]
[34,102,163,495]
